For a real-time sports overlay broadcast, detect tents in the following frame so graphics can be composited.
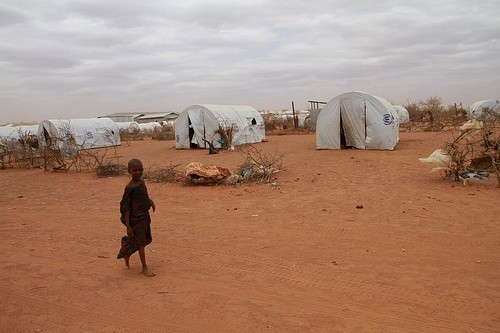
[0,117,176,152]
[258,110,314,129]
[392,104,409,124]
[471,99,500,123]
[315,91,400,151]
[174,104,266,149]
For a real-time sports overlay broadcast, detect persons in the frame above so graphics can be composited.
[116,158,158,278]
